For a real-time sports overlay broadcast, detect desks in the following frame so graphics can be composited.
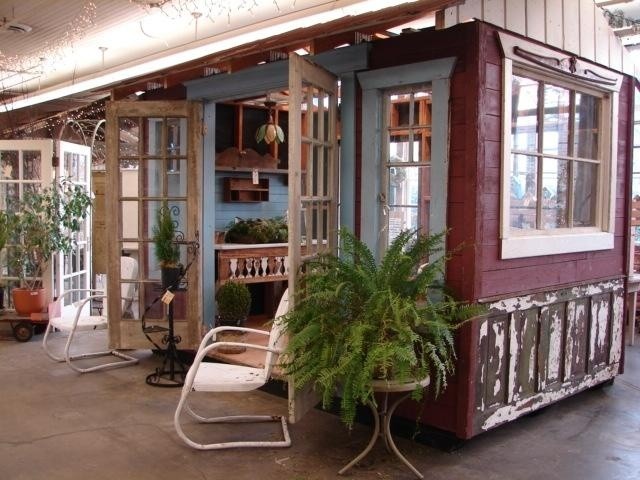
[338,372,431,479]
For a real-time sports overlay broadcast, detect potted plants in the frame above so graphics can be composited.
[0,175,95,316]
[153,191,182,291]
[260,223,493,430]
[216,280,251,327]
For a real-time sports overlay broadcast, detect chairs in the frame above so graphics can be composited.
[42,255,138,373]
[174,288,291,450]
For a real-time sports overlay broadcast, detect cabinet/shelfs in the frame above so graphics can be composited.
[223,177,270,203]
[215,102,308,174]
